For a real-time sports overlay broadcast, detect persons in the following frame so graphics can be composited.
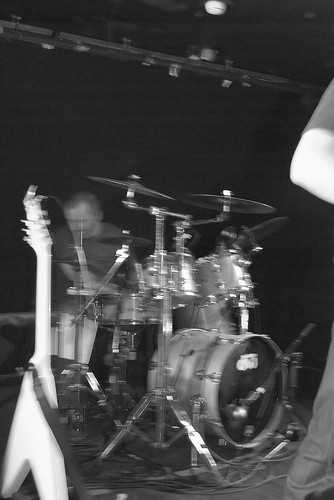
[286,53,334,500]
[48,186,164,398]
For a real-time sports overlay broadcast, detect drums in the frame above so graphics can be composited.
[194,249,256,303]
[96,291,160,327]
[139,251,197,309]
[146,326,288,448]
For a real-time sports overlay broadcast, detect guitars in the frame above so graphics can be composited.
[0,186,73,500]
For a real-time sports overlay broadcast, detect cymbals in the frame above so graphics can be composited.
[182,193,277,215]
[84,175,176,203]
